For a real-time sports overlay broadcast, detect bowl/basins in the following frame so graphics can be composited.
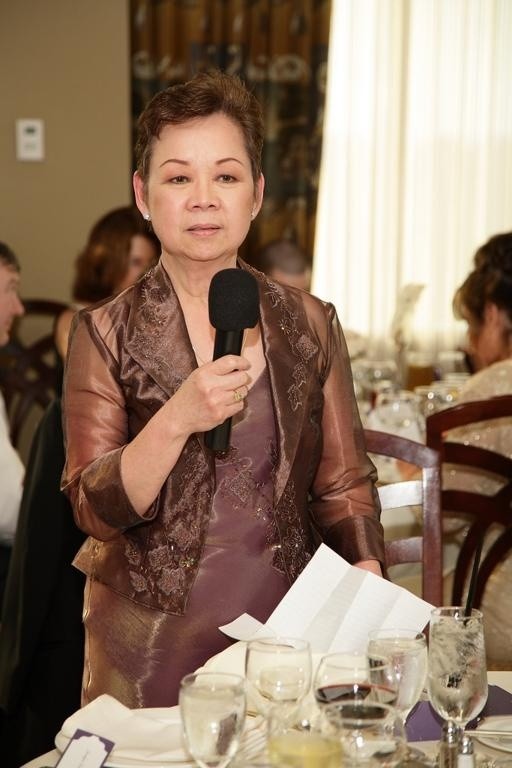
[315,683,398,728]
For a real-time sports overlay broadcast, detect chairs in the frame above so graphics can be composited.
[6,291,79,456]
[331,431,442,641]
[422,394,512,667]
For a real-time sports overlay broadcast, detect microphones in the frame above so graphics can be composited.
[205,268,262,459]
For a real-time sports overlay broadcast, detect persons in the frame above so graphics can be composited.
[385,262,512,671]
[0,204,162,768]
[256,237,312,291]
[62,70,390,710]
[472,233,511,271]
[0,239,27,546]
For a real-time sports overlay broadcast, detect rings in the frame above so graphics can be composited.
[233,389,245,403]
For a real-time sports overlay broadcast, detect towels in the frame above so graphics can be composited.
[59,692,268,766]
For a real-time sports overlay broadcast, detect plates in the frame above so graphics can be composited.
[475,717,512,755]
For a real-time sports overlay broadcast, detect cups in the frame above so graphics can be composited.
[312,654,399,738]
[428,608,490,768]
[179,672,245,768]
[358,361,398,404]
[367,628,426,761]
[313,701,407,767]
[374,392,416,435]
[245,639,309,768]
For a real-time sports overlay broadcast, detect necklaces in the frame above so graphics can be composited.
[192,327,251,365]
